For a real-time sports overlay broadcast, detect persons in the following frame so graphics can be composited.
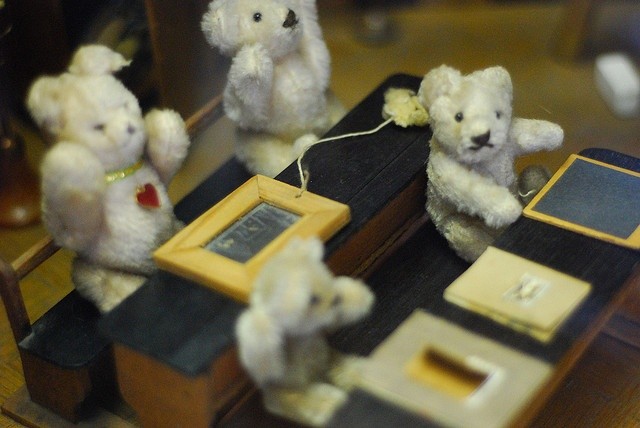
[27,44,191,313]
[415,62,567,262]
[196,0,346,182]
[234,232,375,428]
